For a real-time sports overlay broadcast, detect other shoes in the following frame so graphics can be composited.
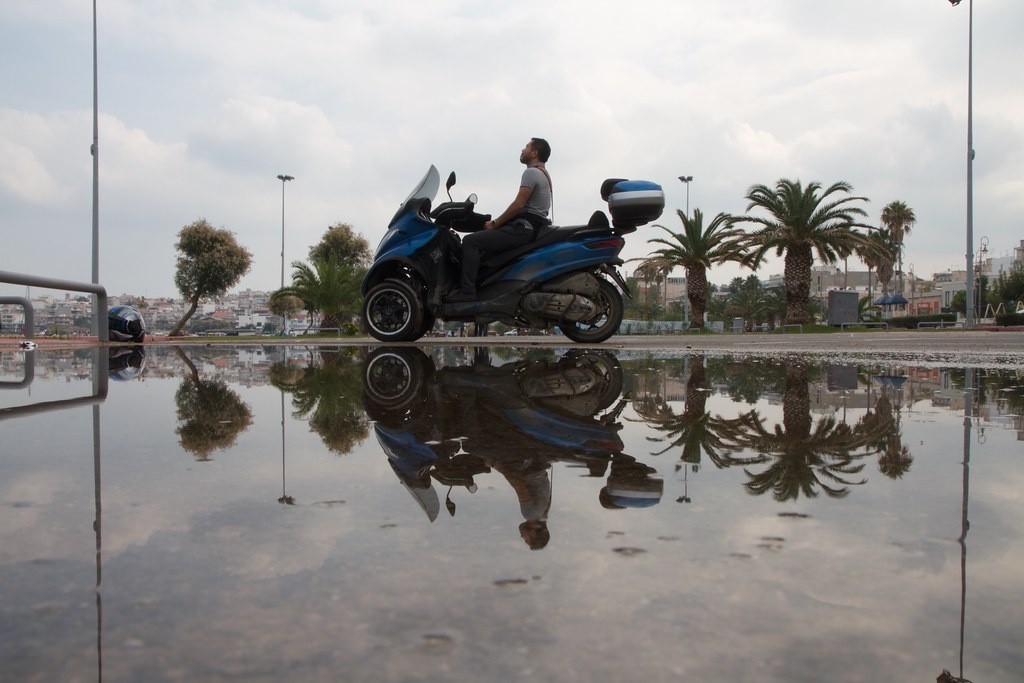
[445,287,477,303]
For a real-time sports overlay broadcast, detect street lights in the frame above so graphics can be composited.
[678,175,693,220]
[276,174,295,336]
[949,0,974,327]
[908,263,914,315]
[277,345,295,505]
[978,236,989,326]
[937,368,975,683]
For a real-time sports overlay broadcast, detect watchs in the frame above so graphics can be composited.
[491,460,495,468]
[492,219,497,228]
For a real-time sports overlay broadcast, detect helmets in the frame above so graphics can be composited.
[107,345,147,380]
[107,305,147,343]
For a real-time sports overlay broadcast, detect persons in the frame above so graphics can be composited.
[446,387,551,550]
[445,138,553,301]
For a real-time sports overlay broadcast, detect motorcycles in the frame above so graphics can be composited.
[361,346,664,523]
[362,163,664,343]
